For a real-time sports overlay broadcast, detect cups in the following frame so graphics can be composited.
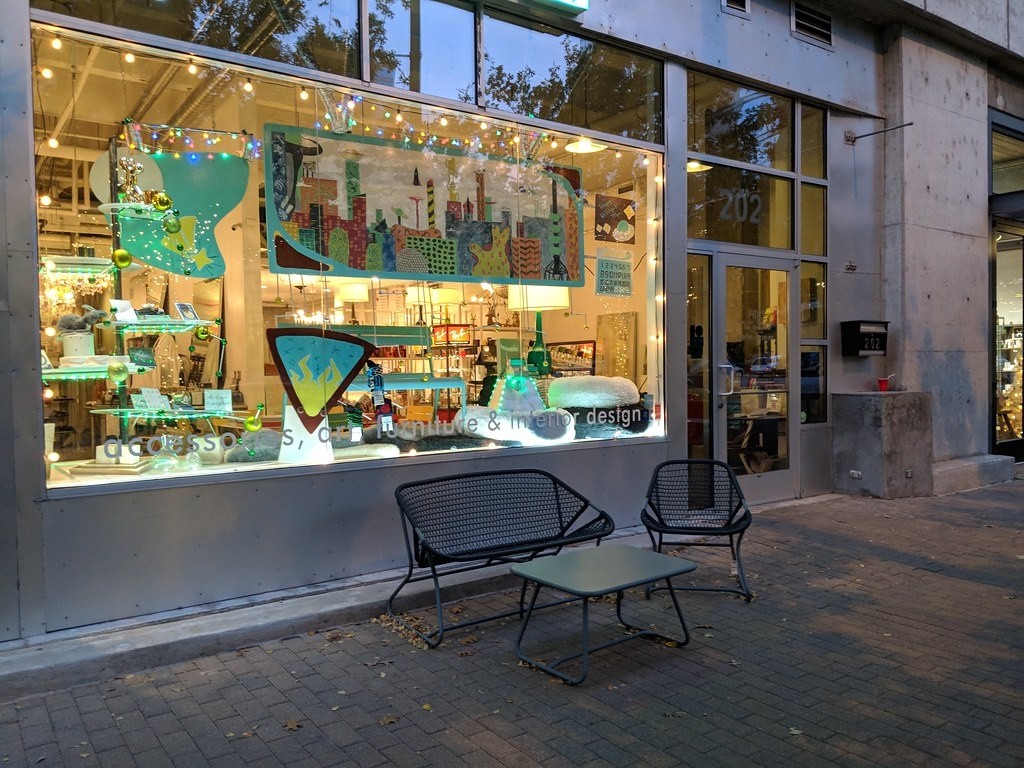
[878,377,888,391]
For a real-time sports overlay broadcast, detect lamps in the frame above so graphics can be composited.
[565,70,609,154]
[687,75,713,172]
[508,284,570,376]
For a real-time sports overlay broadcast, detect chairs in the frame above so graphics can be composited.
[641,460,754,601]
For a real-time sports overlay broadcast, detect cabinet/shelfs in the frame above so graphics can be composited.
[88,320,229,444]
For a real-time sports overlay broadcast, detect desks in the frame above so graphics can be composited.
[510,540,696,685]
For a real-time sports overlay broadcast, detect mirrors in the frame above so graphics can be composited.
[188,275,224,388]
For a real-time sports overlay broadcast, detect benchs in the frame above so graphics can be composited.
[278,324,465,427]
[387,468,616,648]
